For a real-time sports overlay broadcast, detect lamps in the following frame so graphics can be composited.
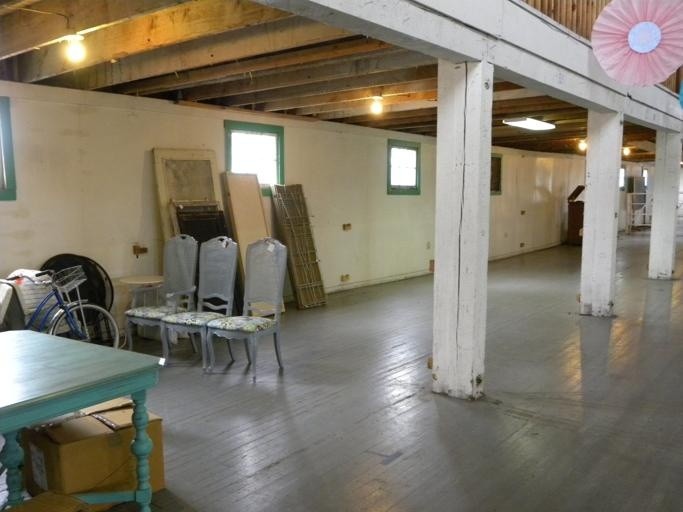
[61,13,88,64]
[502,117,556,131]
[369,87,384,118]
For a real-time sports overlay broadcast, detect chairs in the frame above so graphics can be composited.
[123,234,288,381]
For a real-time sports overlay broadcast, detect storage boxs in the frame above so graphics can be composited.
[1,488,91,512]
[21,396,166,512]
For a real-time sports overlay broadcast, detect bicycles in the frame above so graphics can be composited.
[6,265,119,350]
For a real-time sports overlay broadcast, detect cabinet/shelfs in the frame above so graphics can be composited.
[625,192,653,236]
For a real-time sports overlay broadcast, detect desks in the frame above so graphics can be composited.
[0,328,163,511]
